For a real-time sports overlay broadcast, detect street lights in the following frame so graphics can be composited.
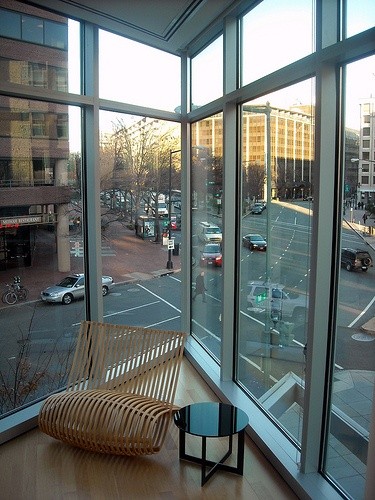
[167,146,207,268]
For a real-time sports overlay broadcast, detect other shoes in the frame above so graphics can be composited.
[202,300,208,303]
[192,297,195,302]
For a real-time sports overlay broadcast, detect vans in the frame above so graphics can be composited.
[198,222,222,244]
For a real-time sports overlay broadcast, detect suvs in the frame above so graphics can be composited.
[240,281,310,328]
[200,243,223,268]
[340,247,372,272]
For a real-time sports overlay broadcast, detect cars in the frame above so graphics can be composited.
[40,273,113,305]
[252,200,267,215]
[242,234,267,252]
[100,189,198,238]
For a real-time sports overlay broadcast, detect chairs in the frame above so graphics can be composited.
[38,319,186,456]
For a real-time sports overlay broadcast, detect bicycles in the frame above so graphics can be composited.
[2,281,27,305]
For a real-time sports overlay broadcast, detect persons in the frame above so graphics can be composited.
[192,271,208,303]
[362,213,367,223]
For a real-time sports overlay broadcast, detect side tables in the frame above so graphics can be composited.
[174,402,249,487]
